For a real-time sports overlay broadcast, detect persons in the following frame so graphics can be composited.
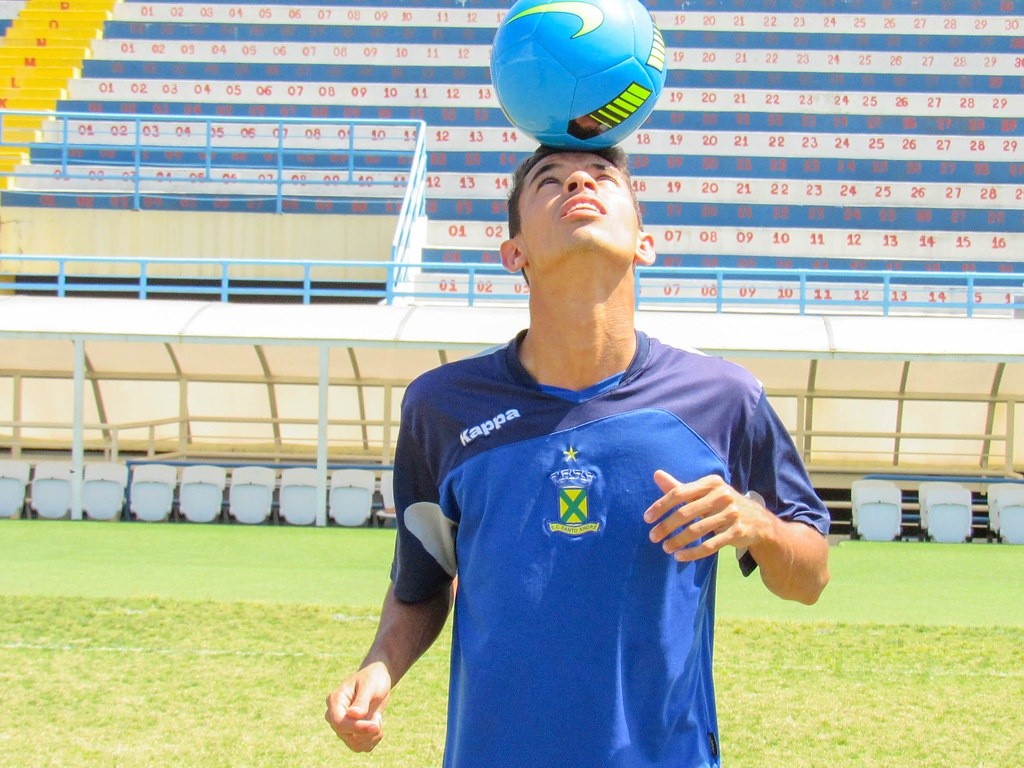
[326,144,831,768]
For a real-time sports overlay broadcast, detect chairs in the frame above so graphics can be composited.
[31,462,72,519]
[988,483,1024,544]
[279,468,317,525]
[918,481,972,542]
[229,466,276,525]
[130,463,177,521]
[82,463,128,521]
[0,460,30,519]
[850,483,902,540]
[179,464,227,523]
[376,471,397,528]
[329,469,375,527]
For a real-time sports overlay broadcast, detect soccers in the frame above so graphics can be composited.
[489,1,671,154]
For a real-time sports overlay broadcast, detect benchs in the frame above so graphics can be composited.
[0,0,1024,309]
